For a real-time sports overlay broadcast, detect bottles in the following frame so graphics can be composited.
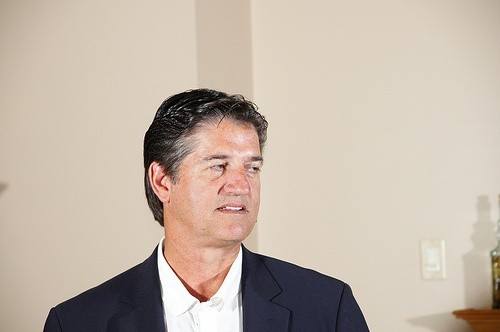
[490,193,500,310]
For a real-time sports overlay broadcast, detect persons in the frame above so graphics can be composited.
[43,88,370,332]
